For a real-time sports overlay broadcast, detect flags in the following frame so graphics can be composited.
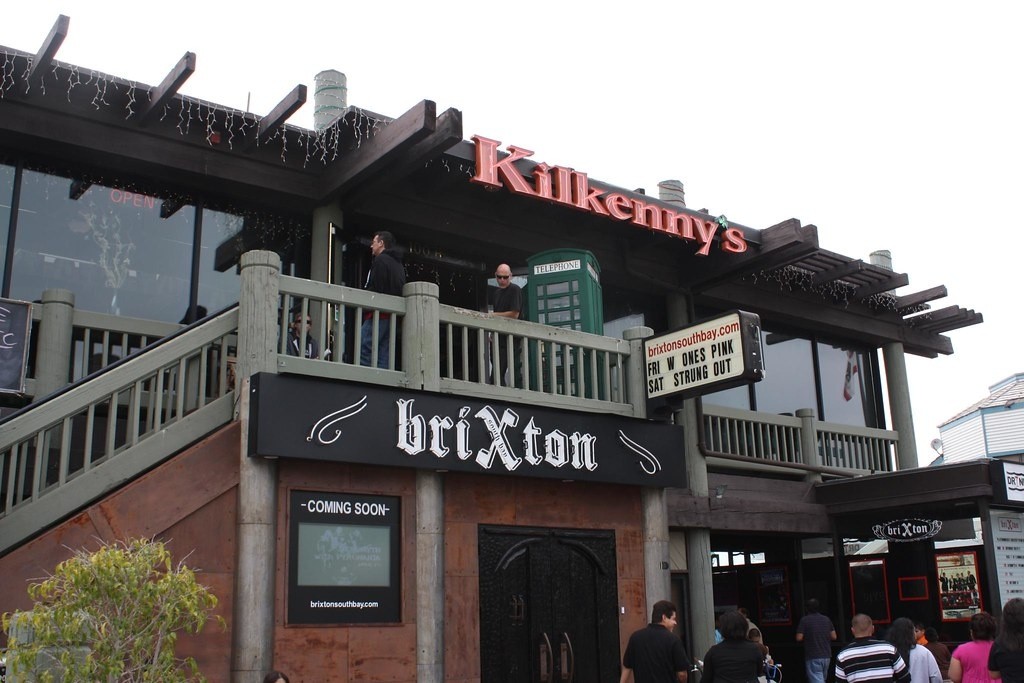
[844,347,858,401]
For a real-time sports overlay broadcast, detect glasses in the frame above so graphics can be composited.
[496,273,510,278]
[296,318,310,324]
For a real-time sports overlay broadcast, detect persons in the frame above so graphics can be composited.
[940,570,978,606]
[797,599,837,683]
[947,613,1002,683]
[987,598,1024,683]
[914,622,928,645]
[887,618,943,683]
[620,600,689,683]
[488,264,523,387]
[835,614,911,683]
[923,628,951,680]
[361,231,401,369]
[699,608,774,683]
[279,313,319,360]
